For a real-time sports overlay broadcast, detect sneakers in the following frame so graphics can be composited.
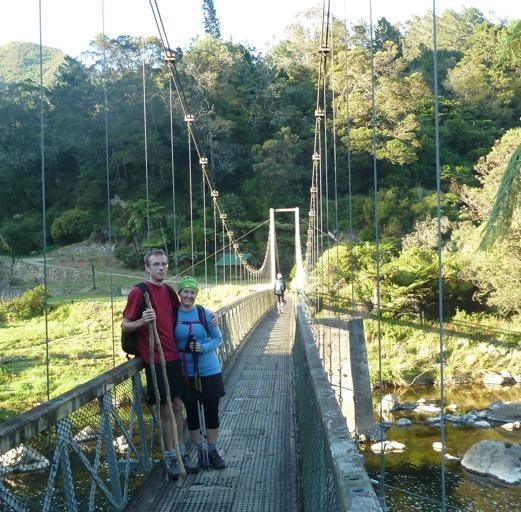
[163,450,180,479]
[181,450,200,475]
[196,449,210,471]
[208,447,226,469]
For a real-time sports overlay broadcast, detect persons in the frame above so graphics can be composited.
[121,249,219,479]
[172,276,226,469]
[274,272,287,314]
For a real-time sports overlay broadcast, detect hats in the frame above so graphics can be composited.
[177,275,202,293]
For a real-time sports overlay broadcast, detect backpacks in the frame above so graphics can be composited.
[119,282,177,357]
[275,280,284,294]
